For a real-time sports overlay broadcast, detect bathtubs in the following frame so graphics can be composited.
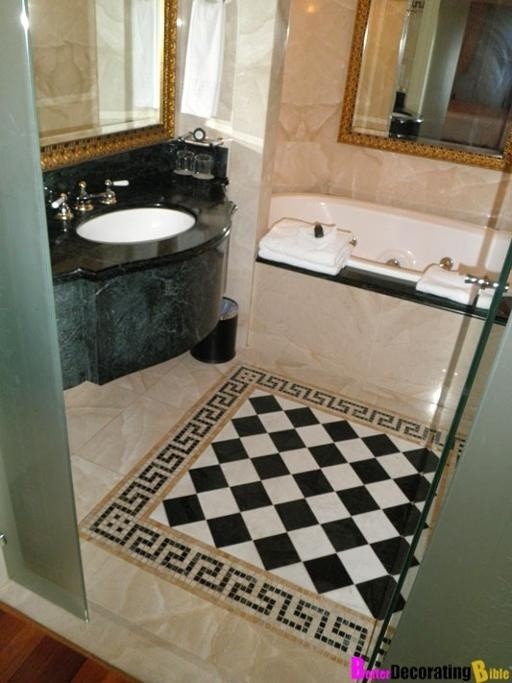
[266,179,512,304]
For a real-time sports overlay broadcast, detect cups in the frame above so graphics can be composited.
[194,152,212,177]
[177,150,194,174]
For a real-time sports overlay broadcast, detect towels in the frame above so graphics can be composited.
[416,264,495,308]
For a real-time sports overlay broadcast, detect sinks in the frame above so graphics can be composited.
[76,203,196,244]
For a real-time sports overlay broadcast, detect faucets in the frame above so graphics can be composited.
[72,181,113,212]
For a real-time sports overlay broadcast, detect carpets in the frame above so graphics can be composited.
[77,358,448,669]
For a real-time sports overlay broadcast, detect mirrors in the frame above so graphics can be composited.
[26,1,179,173]
[337,0,512,172]
